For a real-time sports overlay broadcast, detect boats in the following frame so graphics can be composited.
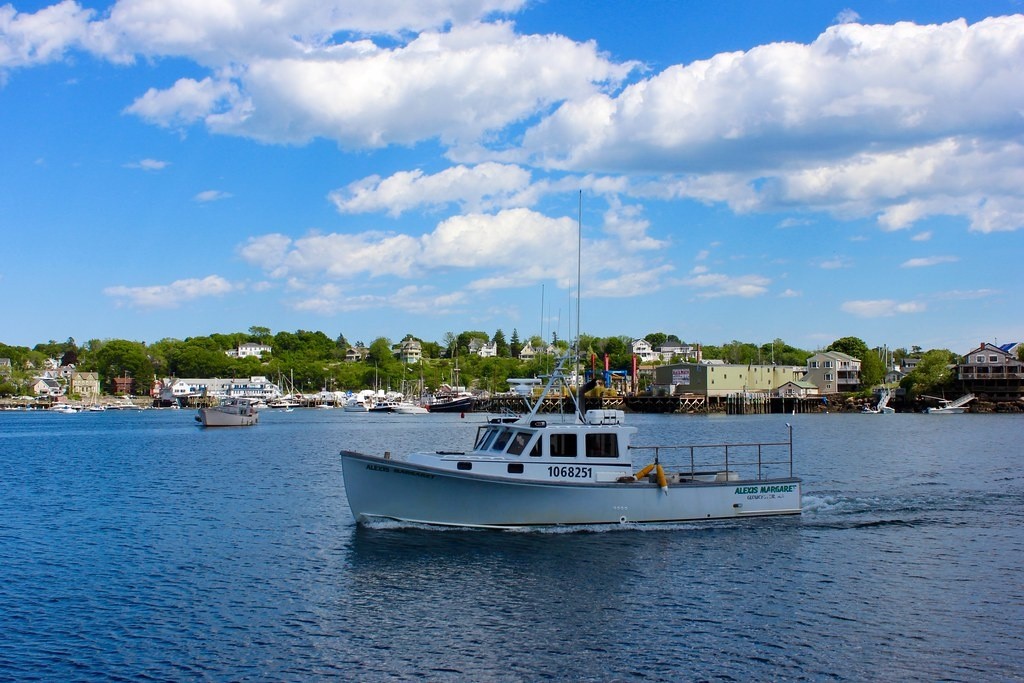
[334,185,823,539]
[928,400,969,416]
[263,357,472,415]
[50,398,182,414]
[195,396,260,427]
